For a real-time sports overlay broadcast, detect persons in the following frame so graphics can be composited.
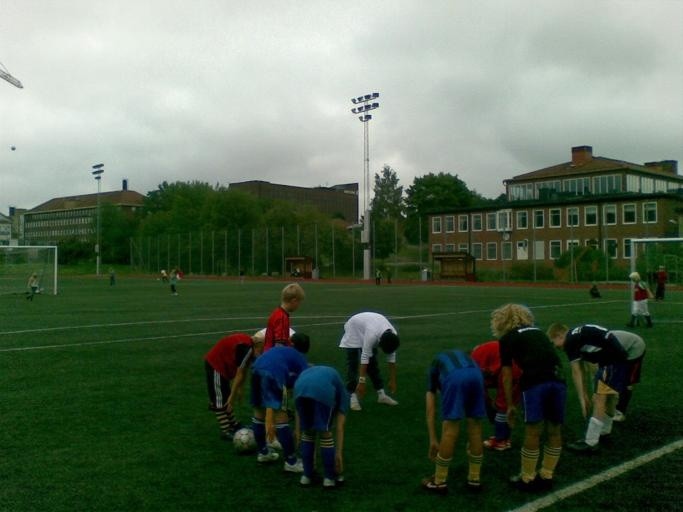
[203,332,265,441]
[174,272,183,280]
[247,332,311,472]
[374,266,385,286]
[262,283,305,351]
[26,274,40,302]
[252,325,310,361]
[490,303,567,493]
[470,339,522,451]
[546,323,627,458]
[608,331,647,423]
[293,364,350,488]
[422,350,487,496]
[107,268,117,287]
[624,271,654,328]
[654,266,668,303]
[169,269,178,297]
[339,312,401,411]
[158,267,168,285]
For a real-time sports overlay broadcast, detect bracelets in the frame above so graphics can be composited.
[358,375,367,384]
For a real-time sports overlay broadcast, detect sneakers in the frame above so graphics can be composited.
[283,458,306,473]
[299,474,313,485]
[505,473,541,496]
[537,475,556,493]
[482,438,505,451]
[257,452,280,462]
[458,478,483,497]
[349,393,362,411]
[504,441,512,449]
[612,410,626,422]
[419,474,448,494]
[376,395,399,406]
[567,440,599,456]
[320,474,347,488]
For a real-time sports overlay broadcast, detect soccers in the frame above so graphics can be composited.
[231,428,257,452]
